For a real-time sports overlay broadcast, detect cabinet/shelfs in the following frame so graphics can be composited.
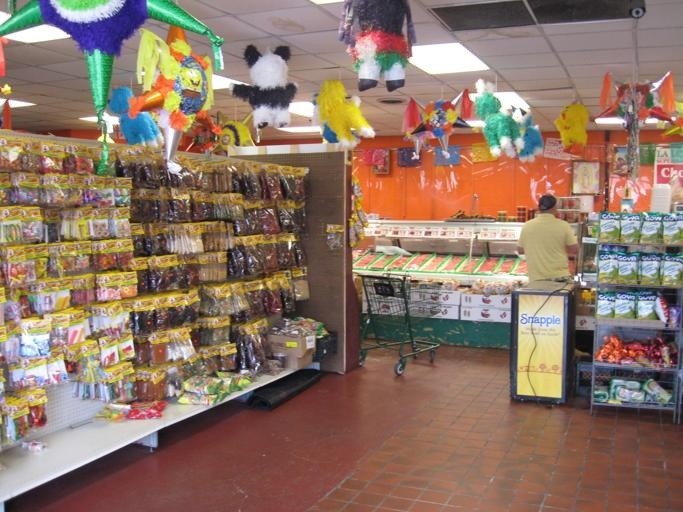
[591,240,683,428]
[575,217,598,330]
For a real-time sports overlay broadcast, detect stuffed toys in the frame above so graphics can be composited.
[476,78,523,158]
[312,81,375,147]
[510,104,543,161]
[338,0,416,93]
[228,45,297,129]
[555,101,591,153]
[106,87,164,147]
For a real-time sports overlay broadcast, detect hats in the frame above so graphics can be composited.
[539,194,556,211]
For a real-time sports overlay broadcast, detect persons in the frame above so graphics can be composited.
[518,194,578,279]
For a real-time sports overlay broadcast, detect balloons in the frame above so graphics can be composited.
[1,0,221,176]
[218,109,254,147]
[126,21,222,173]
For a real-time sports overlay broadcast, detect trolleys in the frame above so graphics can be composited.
[357,271,443,376]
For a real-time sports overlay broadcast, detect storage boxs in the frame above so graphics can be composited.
[266,324,313,370]
[362,277,594,330]
